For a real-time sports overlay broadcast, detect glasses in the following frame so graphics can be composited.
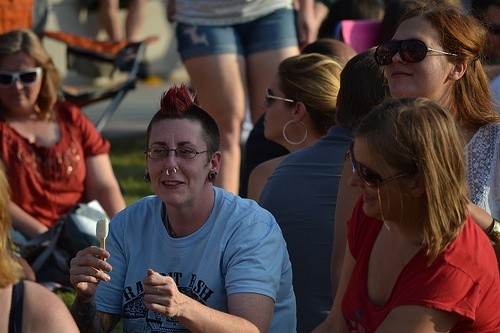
[0,66,42,89]
[348,139,415,188]
[144,146,211,159]
[472,9,500,35]
[264,88,295,108]
[374,39,460,66]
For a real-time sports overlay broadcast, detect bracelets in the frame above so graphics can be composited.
[488,218,500,245]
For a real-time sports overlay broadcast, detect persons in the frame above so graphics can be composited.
[0,1,500,333]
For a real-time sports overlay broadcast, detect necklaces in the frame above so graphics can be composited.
[169,224,176,238]
